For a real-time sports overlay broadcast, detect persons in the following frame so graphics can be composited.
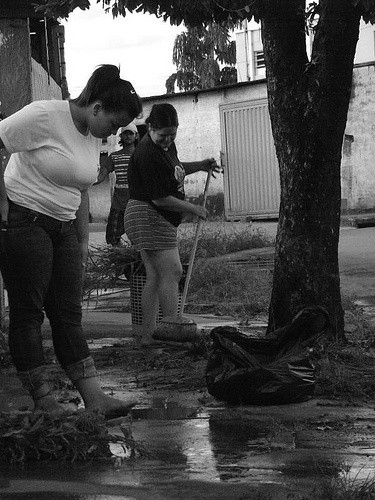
[90,123,141,247]
[0,64,143,420]
[123,103,220,348]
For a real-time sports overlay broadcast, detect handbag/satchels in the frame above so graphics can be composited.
[205,305,329,404]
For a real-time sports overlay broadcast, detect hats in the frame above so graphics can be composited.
[121,123,138,134]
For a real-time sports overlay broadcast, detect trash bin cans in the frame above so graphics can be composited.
[128,262,187,337]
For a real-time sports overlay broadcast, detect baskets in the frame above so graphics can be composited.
[130,264,183,337]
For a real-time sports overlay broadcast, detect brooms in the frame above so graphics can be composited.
[151,169,212,343]
